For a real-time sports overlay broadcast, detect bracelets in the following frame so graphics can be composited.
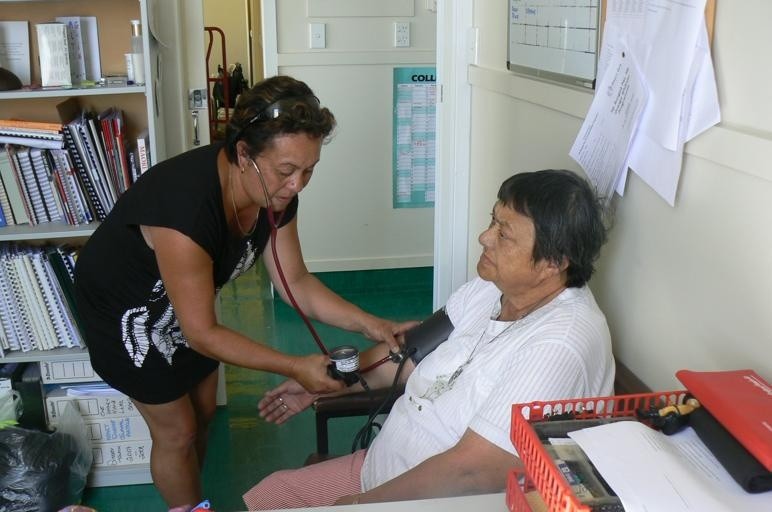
[353,494,360,505]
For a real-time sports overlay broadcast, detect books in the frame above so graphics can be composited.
[0,97,150,227]
[0,242,87,358]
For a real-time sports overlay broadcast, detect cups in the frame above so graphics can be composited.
[124,54,133,81]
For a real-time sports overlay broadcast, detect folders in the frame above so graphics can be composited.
[40,105,132,228]
[0,242,89,353]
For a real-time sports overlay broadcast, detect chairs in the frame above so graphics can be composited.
[316,356,665,458]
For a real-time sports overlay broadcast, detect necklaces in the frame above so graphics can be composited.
[229,165,259,236]
[452,302,541,379]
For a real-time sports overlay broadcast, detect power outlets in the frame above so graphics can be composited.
[394,22,410,48]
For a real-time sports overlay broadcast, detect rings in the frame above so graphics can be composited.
[282,404,288,409]
[279,397,284,403]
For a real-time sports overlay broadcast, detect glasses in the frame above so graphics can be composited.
[237,93,321,138]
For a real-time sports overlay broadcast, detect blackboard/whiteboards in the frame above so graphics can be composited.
[507,0,606,90]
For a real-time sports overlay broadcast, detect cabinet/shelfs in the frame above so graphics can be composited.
[0,1,164,488]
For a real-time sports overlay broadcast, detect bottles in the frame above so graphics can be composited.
[131,19,144,85]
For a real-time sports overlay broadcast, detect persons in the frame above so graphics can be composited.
[73,76,423,511]
[245,171,615,512]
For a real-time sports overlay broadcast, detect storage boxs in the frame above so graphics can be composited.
[506,392,772,512]
